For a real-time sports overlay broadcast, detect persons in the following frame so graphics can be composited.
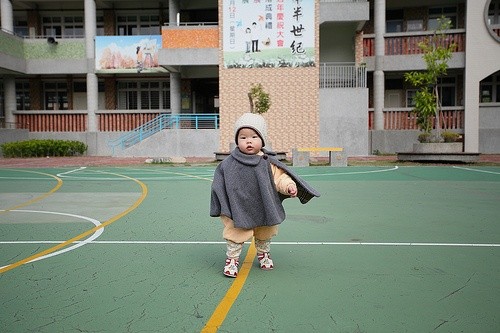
[212,113,297,277]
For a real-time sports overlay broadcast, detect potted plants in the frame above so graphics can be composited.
[418,133,431,143]
[441,132,459,142]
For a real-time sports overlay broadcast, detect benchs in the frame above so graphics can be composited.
[292,148,348,167]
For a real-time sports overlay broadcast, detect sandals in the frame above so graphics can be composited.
[223,258,240,278]
[257,252,274,270]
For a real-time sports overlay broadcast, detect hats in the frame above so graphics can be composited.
[234,112,268,148]
